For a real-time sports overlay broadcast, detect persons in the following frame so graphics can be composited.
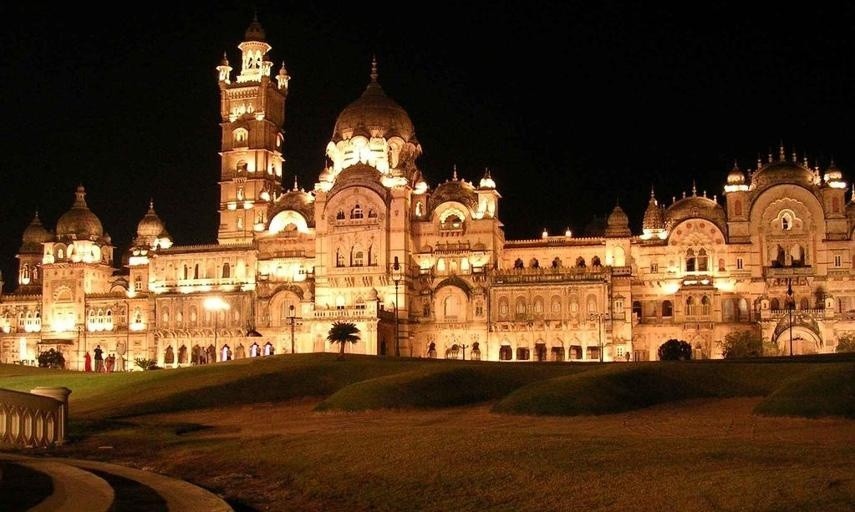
[269,344,275,354]
[255,345,261,356]
[110,354,115,372]
[199,346,206,364]
[84,352,92,372]
[105,354,112,372]
[226,347,233,360]
[93,345,104,372]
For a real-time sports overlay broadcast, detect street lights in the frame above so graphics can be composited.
[391,256,403,356]
[286,305,303,354]
[590,312,609,362]
[786,277,795,356]
